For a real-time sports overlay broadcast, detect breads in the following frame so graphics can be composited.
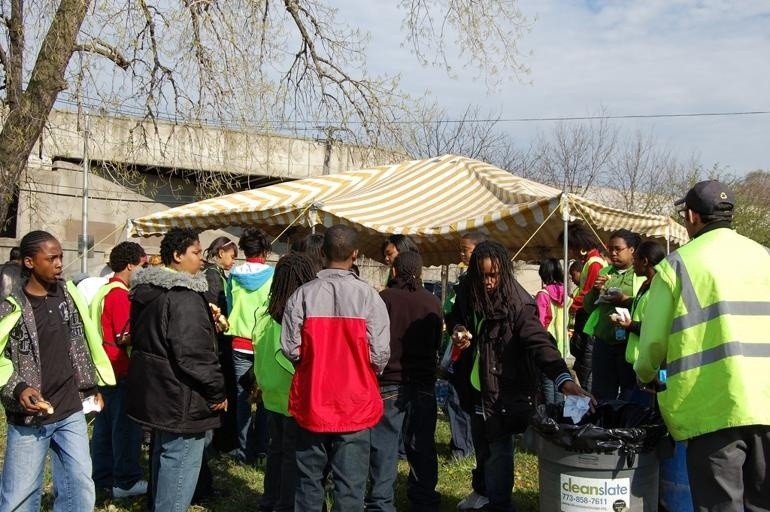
[209,303,220,314]
[35,400,54,417]
[216,315,230,330]
[456,331,473,340]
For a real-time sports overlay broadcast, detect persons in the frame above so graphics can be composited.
[1,224,666,512]
[632,180,770,512]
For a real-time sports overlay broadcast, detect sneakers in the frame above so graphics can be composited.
[112,480,148,498]
[457,492,490,509]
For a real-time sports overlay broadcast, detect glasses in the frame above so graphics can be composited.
[678,208,698,220]
[608,247,628,254]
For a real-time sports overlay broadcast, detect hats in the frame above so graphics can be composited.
[674,180,735,216]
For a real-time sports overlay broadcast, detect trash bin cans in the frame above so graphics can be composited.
[658,433,695,512]
[535,396,678,512]
